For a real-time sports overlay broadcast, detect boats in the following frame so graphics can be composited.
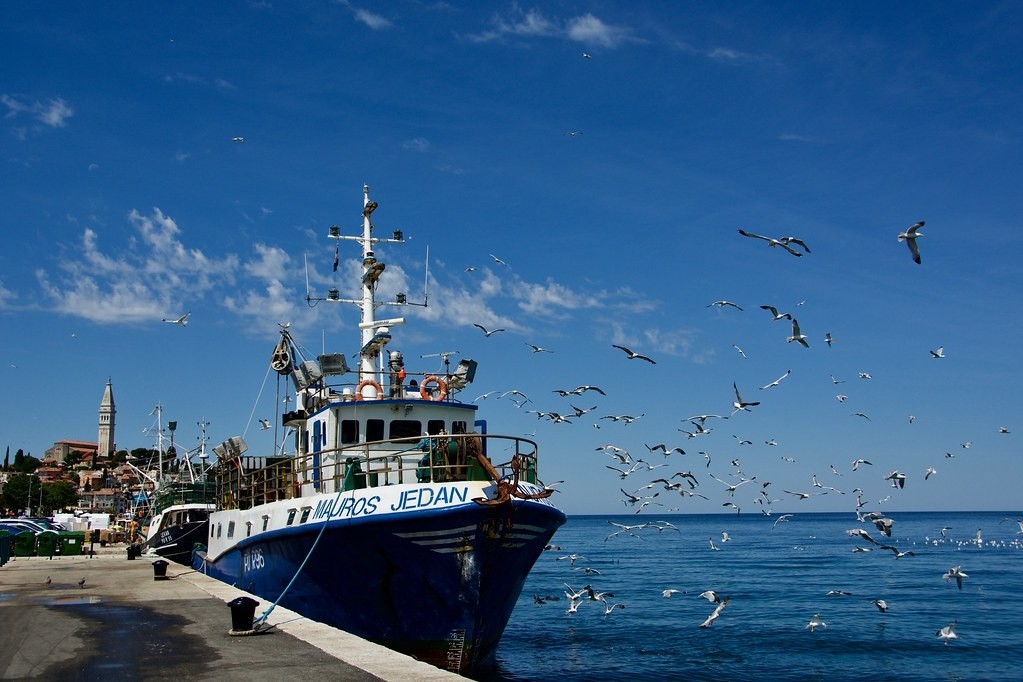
[191,186,568,675]
[145,416,221,566]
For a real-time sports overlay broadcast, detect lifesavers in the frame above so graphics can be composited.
[420,377,447,401]
[355,379,383,401]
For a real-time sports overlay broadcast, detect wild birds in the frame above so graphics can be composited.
[259,419,272,430]
[738,228,811,257]
[787,318,809,349]
[232,137,244,143]
[898,221,925,264]
[563,131,582,136]
[469,340,1009,644]
[761,305,791,321]
[489,254,505,265]
[824,332,832,346]
[161,312,191,326]
[464,267,475,272]
[707,301,743,310]
[77,576,85,588]
[474,323,504,338]
[45,576,51,584]
[796,300,806,307]
[283,395,292,403]
[582,52,590,58]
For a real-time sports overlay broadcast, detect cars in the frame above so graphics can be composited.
[74,508,89,514]
[0,517,66,547]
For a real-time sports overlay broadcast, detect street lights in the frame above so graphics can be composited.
[27,474,35,518]
[39,483,44,518]
